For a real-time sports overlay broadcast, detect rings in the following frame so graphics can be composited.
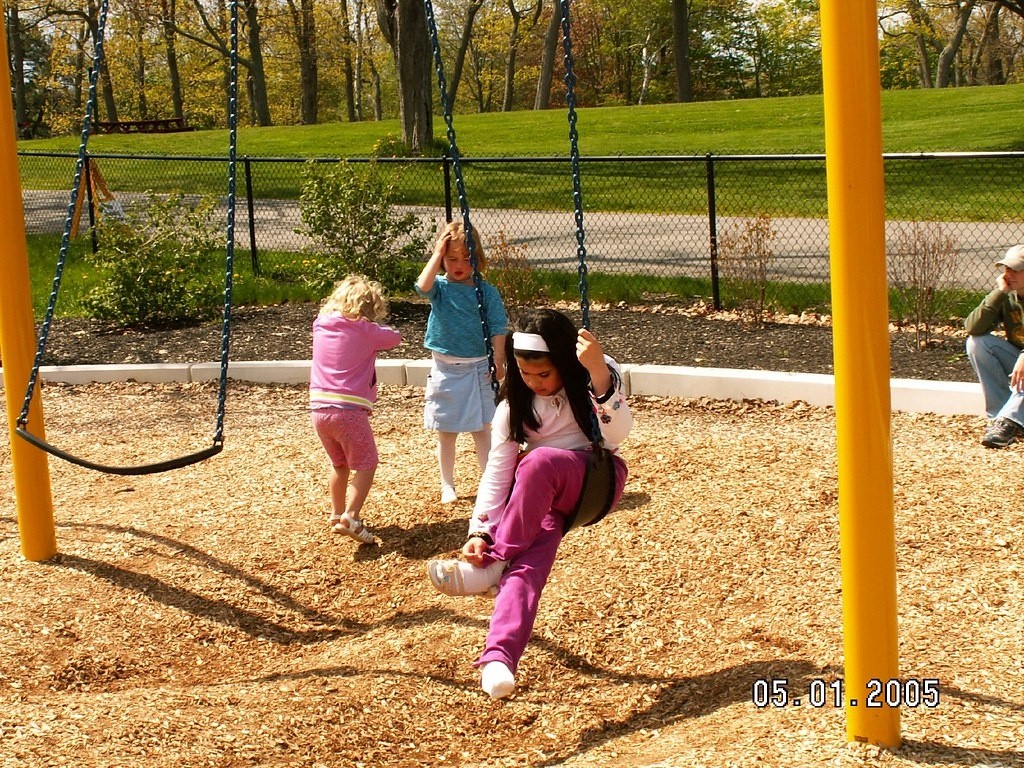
[1021,376,1024,380]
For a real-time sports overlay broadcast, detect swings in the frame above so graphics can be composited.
[16,0,240,477]
[419,1,616,538]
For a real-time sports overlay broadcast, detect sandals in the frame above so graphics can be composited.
[428,554,500,598]
[330,515,340,533]
[334,512,376,545]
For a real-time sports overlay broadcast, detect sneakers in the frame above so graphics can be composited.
[979,416,1024,448]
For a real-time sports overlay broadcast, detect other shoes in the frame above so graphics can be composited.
[440,485,458,504]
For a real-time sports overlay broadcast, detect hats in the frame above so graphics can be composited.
[995,244,1024,271]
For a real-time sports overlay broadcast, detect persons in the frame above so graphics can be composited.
[964,245,1024,448]
[427,309,632,698]
[310,275,400,544]
[415,221,508,504]
